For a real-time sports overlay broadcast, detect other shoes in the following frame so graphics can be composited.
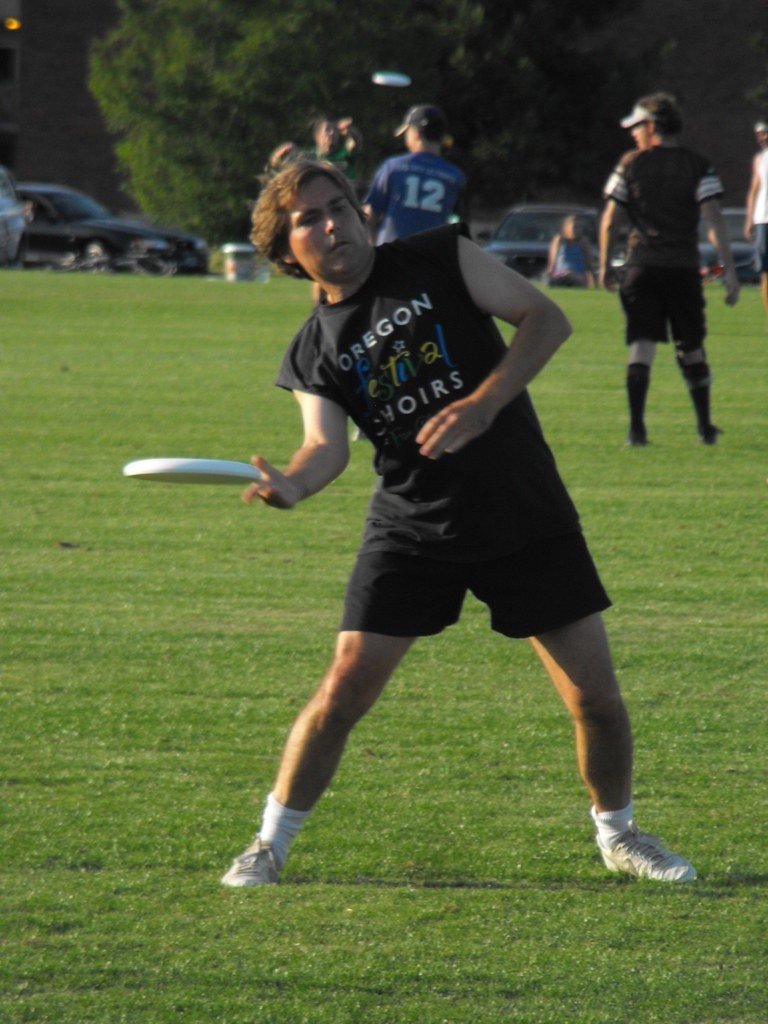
[625,431,647,447]
[698,425,721,445]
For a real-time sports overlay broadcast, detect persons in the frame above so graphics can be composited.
[220,167,698,888]
[271,93,768,449]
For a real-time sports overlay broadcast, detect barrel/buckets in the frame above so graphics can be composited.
[223,242,257,283]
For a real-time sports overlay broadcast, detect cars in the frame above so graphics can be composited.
[0,166,29,268]
[485,204,622,288]
[17,181,211,278]
[698,208,763,283]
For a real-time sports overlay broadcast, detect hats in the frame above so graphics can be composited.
[621,104,664,129]
[395,104,445,138]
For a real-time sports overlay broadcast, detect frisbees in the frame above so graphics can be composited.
[120,457,262,483]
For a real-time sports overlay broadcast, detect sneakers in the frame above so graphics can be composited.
[596,819,697,883]
[220,835,284,889]
